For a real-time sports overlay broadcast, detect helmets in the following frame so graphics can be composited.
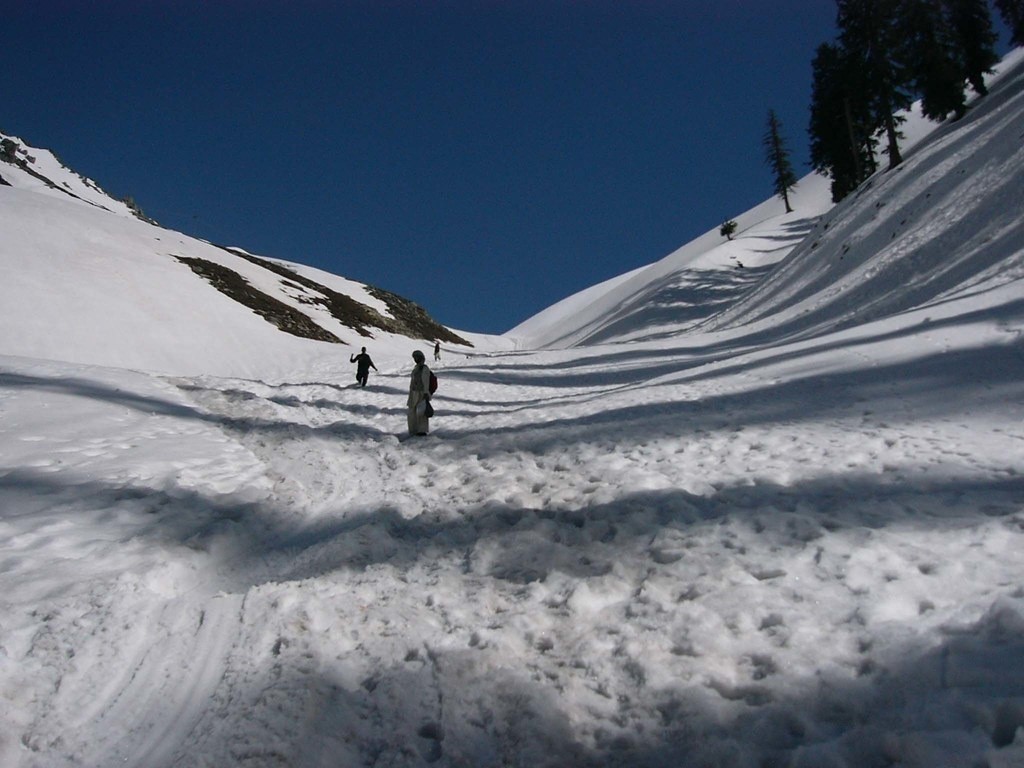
[412,350,425,361]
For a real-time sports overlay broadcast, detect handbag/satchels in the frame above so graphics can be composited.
[416,394,434,418]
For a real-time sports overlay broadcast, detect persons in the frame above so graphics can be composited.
[435,342,441,360]
[350,347,378,386]
[407,350,432,435]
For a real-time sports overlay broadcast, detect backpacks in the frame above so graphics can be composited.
[420,365,437,395]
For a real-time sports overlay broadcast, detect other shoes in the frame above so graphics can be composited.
[417,432,427,437]
